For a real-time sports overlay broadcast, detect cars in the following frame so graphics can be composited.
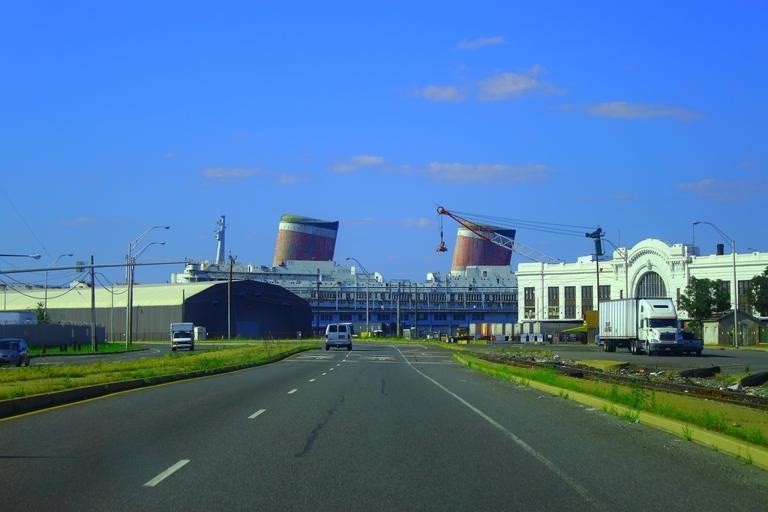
[0,339,30,367]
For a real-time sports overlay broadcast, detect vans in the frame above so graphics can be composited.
[325,323,352,350]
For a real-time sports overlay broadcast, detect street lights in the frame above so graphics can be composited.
[345,257,369,334]
[588,237,628,300]
[126,226,168,348]
[0,254,41,260]
[45,254,73,324]
[693,221,739,348]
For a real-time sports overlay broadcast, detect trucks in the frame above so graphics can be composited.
[170,323,195,352]
[599,297,684,356]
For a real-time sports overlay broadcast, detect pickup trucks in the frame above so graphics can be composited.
[671,331,703,354]
